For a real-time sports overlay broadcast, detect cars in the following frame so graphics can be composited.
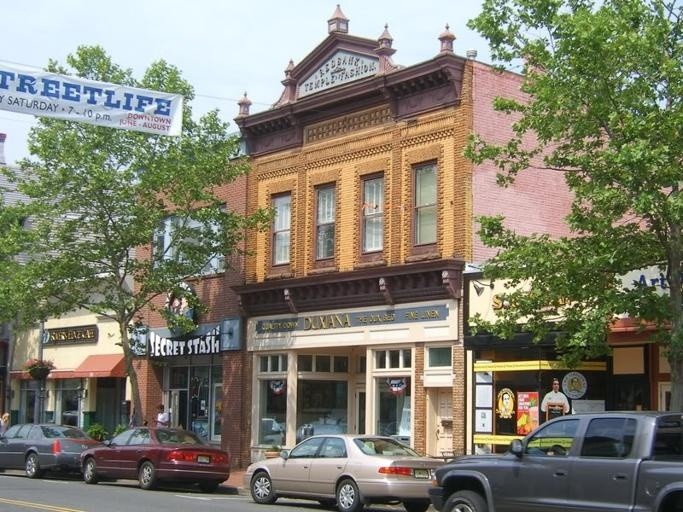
[246,435,446,511]
[0,421,95,479]
[80,425,231,489]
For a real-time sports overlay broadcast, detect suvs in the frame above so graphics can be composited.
[260,419,284,444]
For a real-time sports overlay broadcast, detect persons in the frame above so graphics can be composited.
[0,412,10,439]
[502,392,511,413]
[540,377,570,413]
[572,377,580,391]
[152,404,170,429]
[129,408,135,428]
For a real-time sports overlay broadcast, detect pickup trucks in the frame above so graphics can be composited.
[428,409,683,512]
